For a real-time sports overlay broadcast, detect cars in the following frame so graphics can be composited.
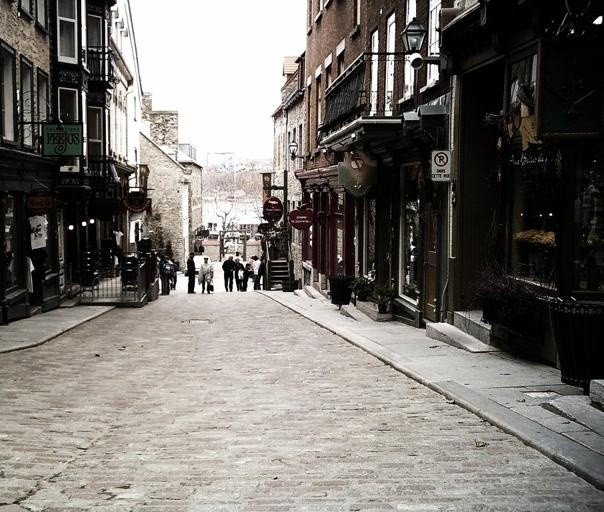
[154,255,173,278]
[180,255,215,276]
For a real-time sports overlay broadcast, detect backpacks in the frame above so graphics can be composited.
[164,263,174,278]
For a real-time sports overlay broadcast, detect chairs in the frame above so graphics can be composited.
[121,271,139,301]
[80,271,100,300]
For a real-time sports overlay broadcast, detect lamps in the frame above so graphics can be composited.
[351,151,365,170]
[400,17,441,65]
[288,140,304,160]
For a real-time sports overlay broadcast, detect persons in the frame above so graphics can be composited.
[186,251,196,293]
[221,255,264,292]
[197,256,214,294]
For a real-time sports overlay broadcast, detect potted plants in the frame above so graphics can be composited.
[367,285,396,314]
[350,276,377,301]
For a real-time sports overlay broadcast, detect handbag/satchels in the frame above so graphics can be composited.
[207,285,213,291]
[238,269,244,280]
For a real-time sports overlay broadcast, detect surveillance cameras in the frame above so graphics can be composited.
[408,53,424,69]
